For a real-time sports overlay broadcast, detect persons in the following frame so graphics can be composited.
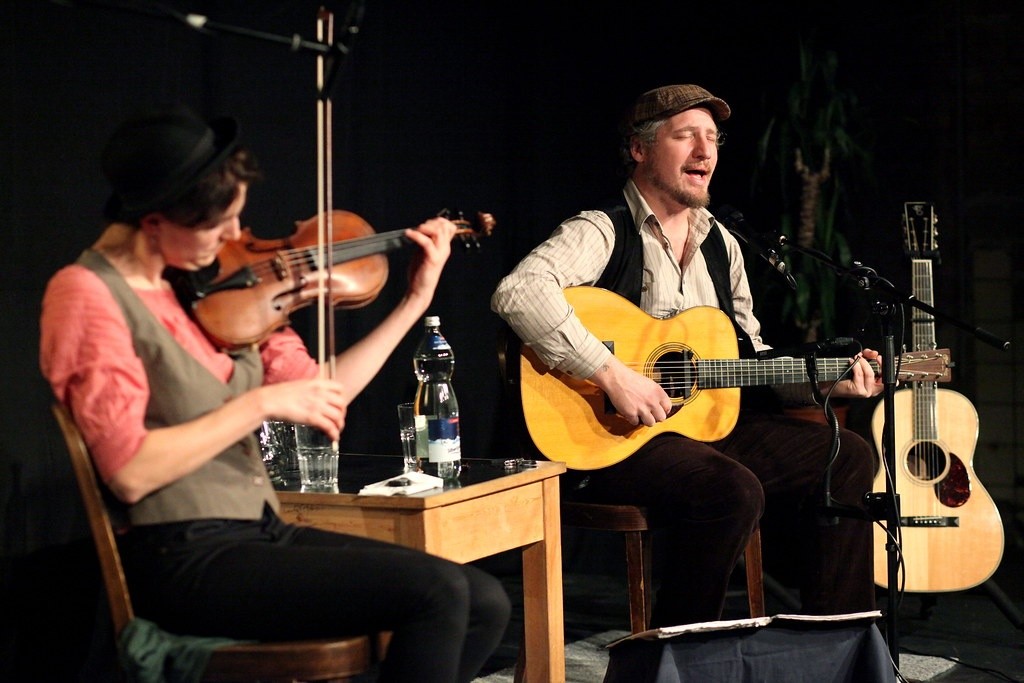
[490,84,885,629]
[40,112,509,683]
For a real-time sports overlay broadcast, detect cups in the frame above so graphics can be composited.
[398,403,416,468]
[294,423,339,491]
[287,424,299,471]
[260,420,288,482]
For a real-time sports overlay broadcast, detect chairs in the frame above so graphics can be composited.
[48,397,394,683]
[494,323,765,634]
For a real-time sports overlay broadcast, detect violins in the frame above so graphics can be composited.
[178,204,499,350]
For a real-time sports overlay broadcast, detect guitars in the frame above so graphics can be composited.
[846,200,1005,593]
[499,285,952,481]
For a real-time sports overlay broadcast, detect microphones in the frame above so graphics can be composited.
[319,0,365,101]
[717,204,797,286]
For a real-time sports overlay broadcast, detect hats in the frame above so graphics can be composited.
[631,84,731,124]
[101,114,244,221]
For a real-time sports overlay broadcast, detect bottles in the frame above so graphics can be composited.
[414,316,462,482]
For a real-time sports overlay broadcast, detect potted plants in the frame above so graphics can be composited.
[754,36,874,432]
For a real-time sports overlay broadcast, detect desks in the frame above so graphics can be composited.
[267,453,568,683]
